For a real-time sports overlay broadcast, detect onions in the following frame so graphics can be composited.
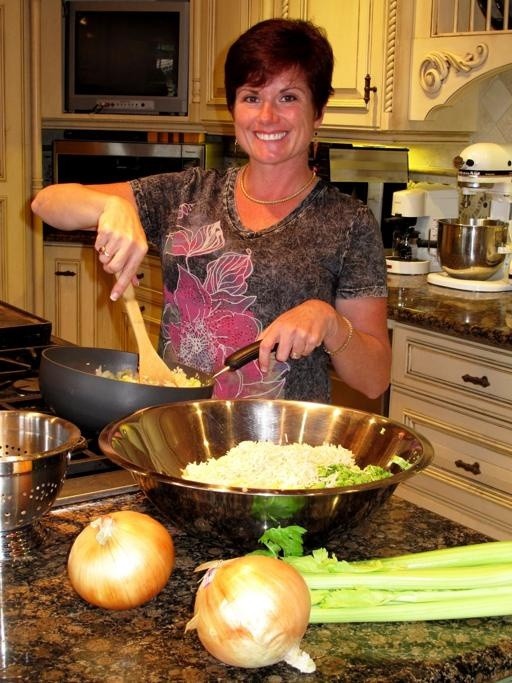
[185,554,318,673]
[67,508,173,610]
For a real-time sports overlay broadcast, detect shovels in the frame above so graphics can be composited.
[101,246,179,388]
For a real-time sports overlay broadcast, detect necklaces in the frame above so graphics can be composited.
[232,164,319,203]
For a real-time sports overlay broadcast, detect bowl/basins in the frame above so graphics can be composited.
[97,393,436,553]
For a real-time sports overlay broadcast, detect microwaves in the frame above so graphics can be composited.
[47,140,205,232]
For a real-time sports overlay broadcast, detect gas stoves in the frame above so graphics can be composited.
[0,344,129,479]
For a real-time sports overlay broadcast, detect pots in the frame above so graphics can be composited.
[35,333,287,441]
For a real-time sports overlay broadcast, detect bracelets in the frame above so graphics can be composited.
[322,312,353,355]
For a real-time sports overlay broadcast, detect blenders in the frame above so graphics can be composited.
[426,140,512,294]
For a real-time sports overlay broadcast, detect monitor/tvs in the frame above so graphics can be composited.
[65,0,189,117]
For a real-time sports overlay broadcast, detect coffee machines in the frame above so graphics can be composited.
[382,187,431,276]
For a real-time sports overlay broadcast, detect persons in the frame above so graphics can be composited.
[28,17,392,405]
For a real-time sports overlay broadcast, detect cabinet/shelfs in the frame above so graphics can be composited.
[1,0,35,317]
[387,324,512,542]
[43,247,122,352]
[200,0,484,132]
[123,254,163,356]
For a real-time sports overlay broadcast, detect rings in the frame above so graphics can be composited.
[97,247,112,259]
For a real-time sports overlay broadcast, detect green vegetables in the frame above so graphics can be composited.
[243,525,512,623]
